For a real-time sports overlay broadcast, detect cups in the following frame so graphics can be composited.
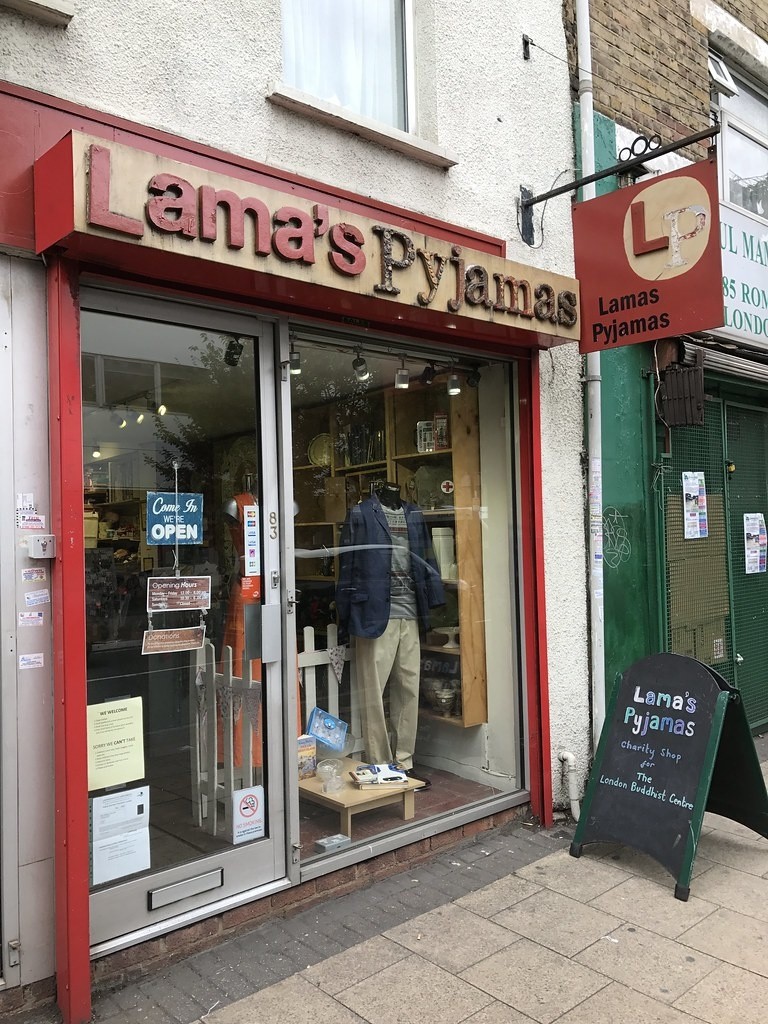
[317,759,345,796]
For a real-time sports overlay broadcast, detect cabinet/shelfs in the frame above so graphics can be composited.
[82,441,175,592]
[292,370,488,729]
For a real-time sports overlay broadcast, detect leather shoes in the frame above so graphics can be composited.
[404,769,431,789]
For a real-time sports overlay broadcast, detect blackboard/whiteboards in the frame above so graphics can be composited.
[567,651,728,885]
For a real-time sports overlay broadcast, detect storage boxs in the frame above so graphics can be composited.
[433,412,449,451]
[305,706,348,754]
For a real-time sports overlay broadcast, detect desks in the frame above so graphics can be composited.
[299,757,426,842]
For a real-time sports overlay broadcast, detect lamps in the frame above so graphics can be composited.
[92,439,101,458]
[109,405,127,429]
[421,360,437,385]
[290,341,301,375]
[124,399,145,425]
[466,368,481,387]
[395,353,410,389]
[447,361,461,396]
[224,334,244,367]
[352,344,370,381]
[145,390,167,416]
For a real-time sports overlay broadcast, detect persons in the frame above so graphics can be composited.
[223,478,300,767]
[336,481,447,789]
